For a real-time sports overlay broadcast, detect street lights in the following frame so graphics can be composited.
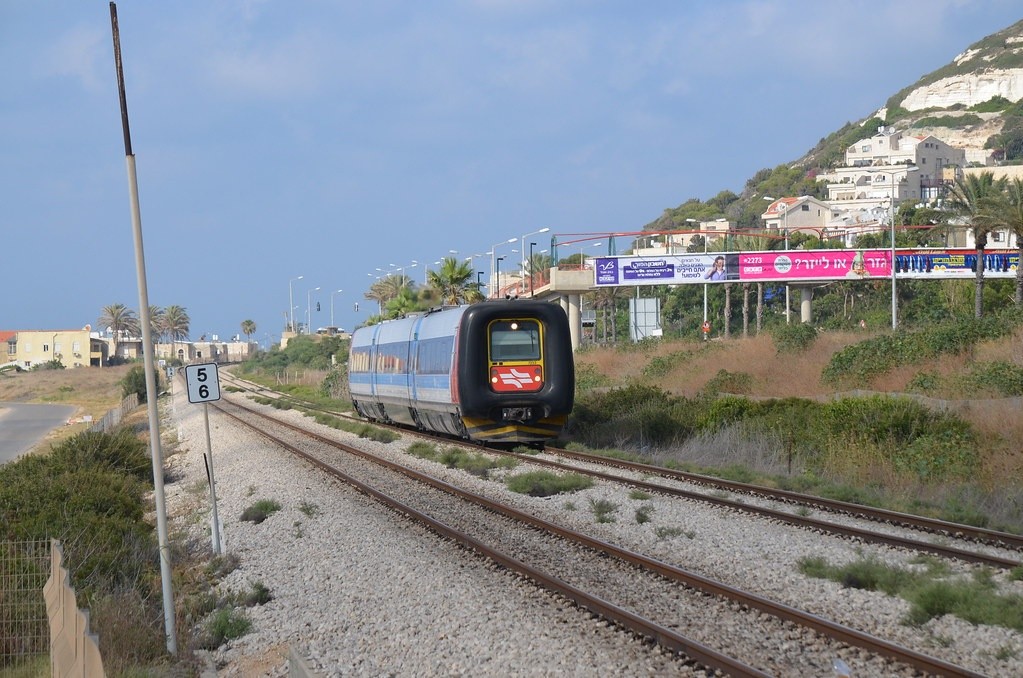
[274,226,551,340]
[630,233,661,296]
[685,218,727,342]
[559,243,603,315]
[51,333,57,362]
[865,166,920,330]
[761,197,808,325]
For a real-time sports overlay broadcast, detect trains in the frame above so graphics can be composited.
[346,294,576,450]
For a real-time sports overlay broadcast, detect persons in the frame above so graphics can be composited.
[704,256,726,281]
[846,252,870,277]
